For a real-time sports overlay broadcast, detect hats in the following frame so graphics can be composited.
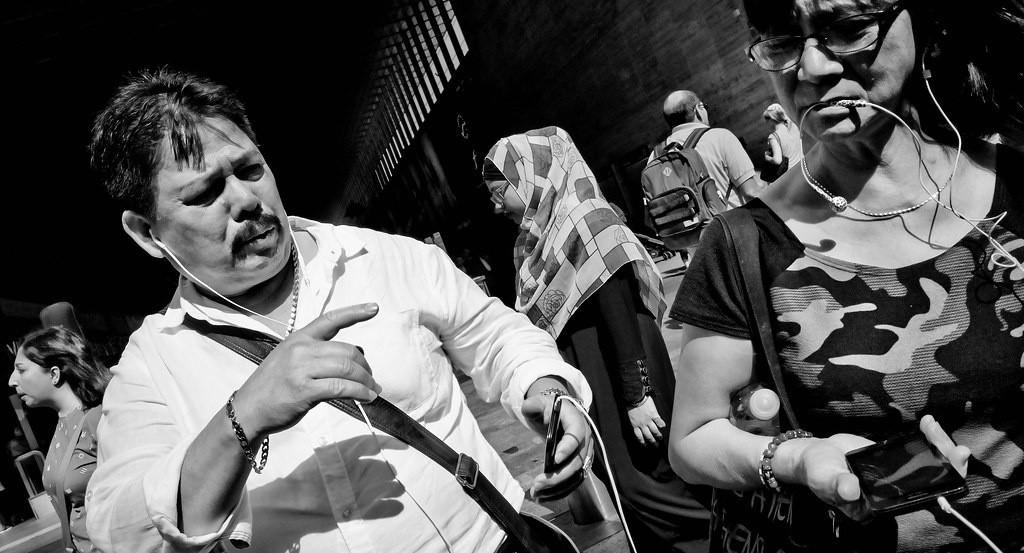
[481,158,506,181]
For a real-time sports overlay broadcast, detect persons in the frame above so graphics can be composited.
[6,325,115,553]
[763,103,804,165]
[669,0,1024,553]
[481,127,713,553]
[84,64,595,553]
[641,90,762,269]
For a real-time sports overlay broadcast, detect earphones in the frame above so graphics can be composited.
[829,196,848,212]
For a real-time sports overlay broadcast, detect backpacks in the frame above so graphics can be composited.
[640,127,733,251]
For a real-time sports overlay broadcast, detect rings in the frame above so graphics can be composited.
[583,456,592,479]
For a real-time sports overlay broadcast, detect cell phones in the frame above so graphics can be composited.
[545,394,562,473]
[846,431,968,516]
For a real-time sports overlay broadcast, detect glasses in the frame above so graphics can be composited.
[747,0,908,72]
[490,181,510,205]
[693,103,710,111]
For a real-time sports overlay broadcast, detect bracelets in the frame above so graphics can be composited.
[227,391,269,474]
[757,429,812,494]
[637,395,647,406]
[540,387,588,414]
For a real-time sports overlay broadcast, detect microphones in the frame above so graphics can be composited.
[148,229,166,248]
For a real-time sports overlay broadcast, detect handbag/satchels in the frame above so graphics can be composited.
[706,208,898,553]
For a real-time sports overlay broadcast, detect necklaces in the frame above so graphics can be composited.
[284,236,299,338]
